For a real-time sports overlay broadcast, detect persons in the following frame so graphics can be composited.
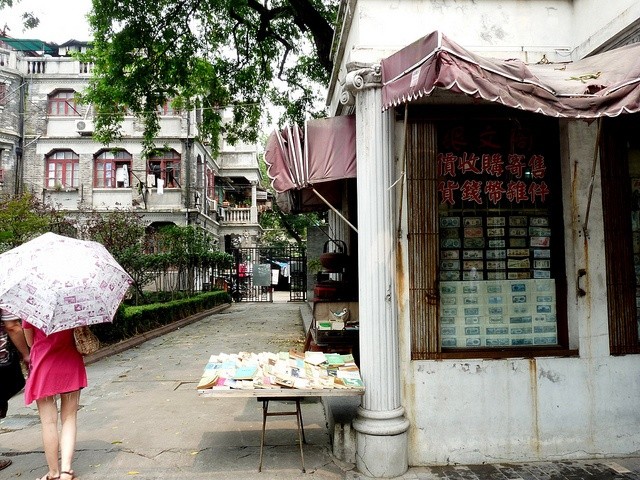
[0,308,31,469]
[21,319,87,480]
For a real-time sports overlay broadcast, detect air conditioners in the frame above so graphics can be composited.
[210,200,217,212]
[75,120,93,135]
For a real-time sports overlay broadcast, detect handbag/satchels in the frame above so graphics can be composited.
[74,325,101,356]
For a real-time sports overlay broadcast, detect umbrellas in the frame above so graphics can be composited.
[0,231,135,337]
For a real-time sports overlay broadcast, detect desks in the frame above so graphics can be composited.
[198,353,365,473]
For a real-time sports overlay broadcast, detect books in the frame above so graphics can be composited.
[196,351,366,391]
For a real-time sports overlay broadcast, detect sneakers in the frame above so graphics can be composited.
[60,470,74,480]
[0,459,12,470]
[35,473,59,480]
